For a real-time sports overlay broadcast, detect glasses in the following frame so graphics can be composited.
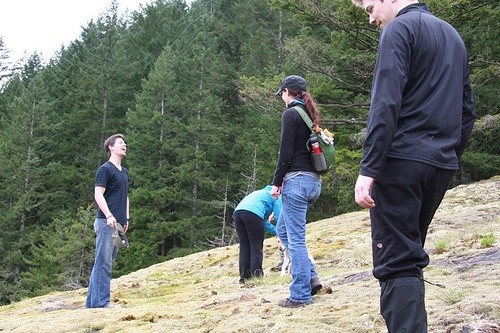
[278,91,284,96]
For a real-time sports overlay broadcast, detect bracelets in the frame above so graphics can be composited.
[107,215,113,219]
[127,219,129,221]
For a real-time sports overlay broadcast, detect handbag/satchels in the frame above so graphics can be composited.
[311,124,336,175]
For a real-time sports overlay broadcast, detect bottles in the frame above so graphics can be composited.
[310,134,328,172]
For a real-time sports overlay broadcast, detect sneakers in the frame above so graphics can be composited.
[278,298,309,308]
[310,279,322,296]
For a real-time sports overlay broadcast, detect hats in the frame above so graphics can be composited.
[112,222,130,249]
[274,75,306,96]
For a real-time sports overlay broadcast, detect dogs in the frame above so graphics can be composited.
[276,235,315,279]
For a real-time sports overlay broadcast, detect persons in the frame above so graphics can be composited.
[86,134,131,308]
[234,185,283,283]
[269,75,323,309]
[353,0,475,333]
